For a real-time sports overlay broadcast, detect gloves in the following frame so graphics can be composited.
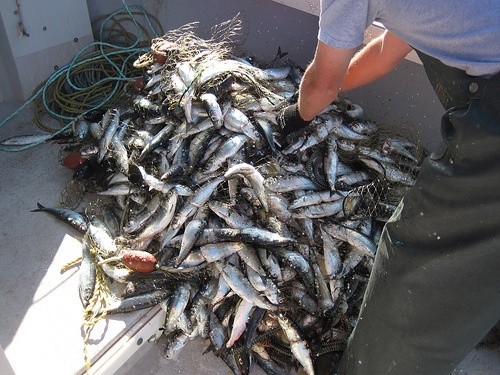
[275,90,312,137]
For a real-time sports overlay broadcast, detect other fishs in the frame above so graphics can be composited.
[0,33,418,375]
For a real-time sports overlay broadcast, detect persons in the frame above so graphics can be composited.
[274,0,500,375]
